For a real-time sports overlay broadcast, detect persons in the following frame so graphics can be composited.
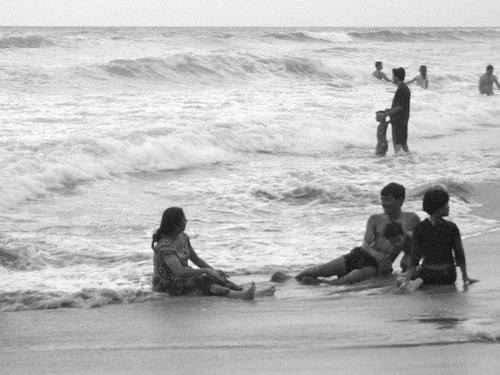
[272,181,422,291]
[375,111,388,157]
[394,185,480,293]
[478,64,500,96]
[405,65,429,89]
[371,61,392,82]
[385,67,410,155]
[151,207,276,301]
